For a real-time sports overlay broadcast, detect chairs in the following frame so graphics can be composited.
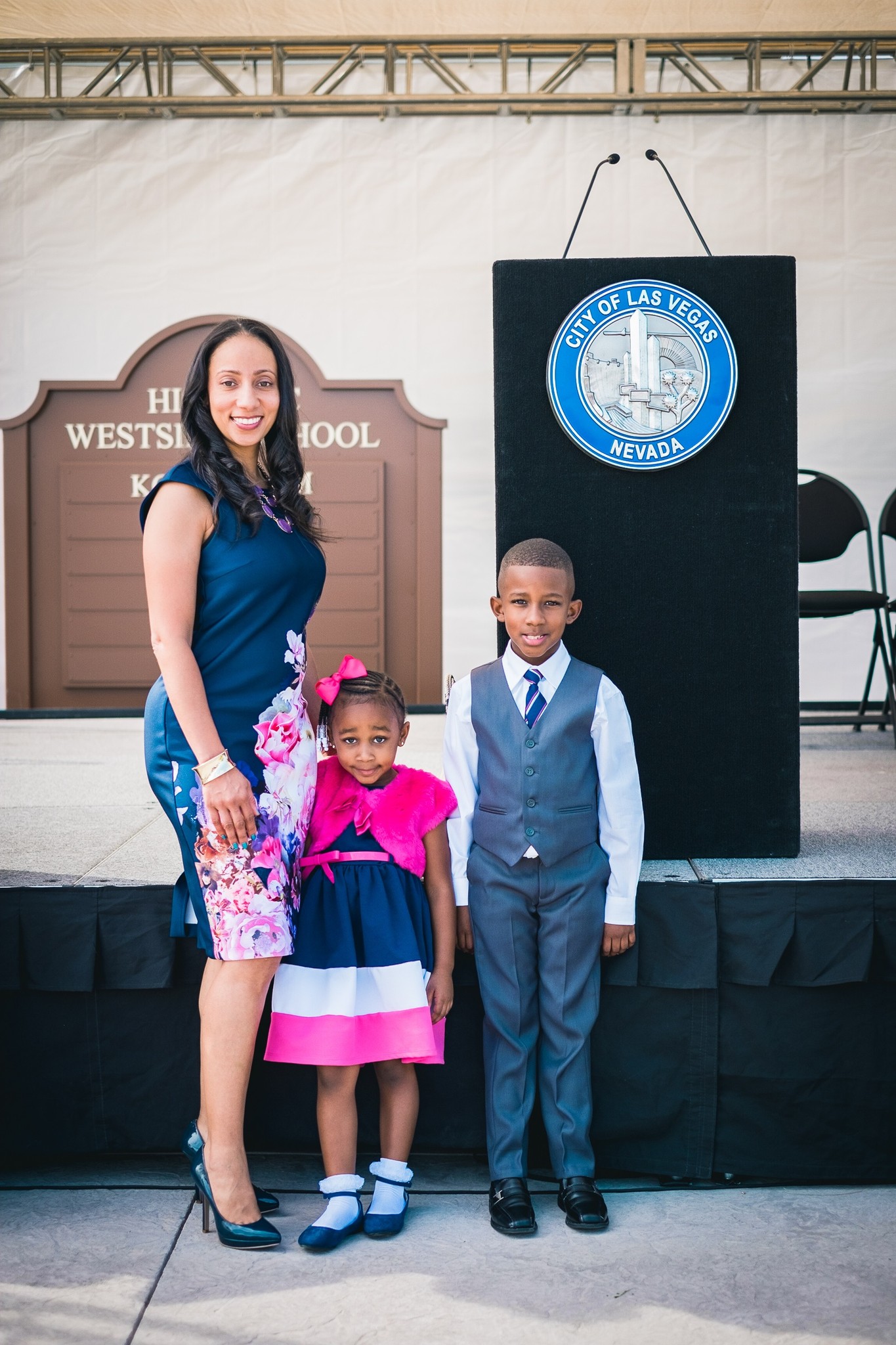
[797,468,896,744]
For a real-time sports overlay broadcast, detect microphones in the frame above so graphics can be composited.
[645,148,714,257]
[562,152,620,258]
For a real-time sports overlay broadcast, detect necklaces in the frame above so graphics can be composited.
[250,461,294,532]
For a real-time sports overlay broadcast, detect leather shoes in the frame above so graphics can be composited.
[489,1176,537,1235]
[558,1176,609,1229]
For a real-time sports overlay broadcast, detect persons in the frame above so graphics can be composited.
[262,655,459,1250]
[443,539,645,1233]
[138,317,334,1249]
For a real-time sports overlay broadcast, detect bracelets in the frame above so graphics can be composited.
[192,749,237,785]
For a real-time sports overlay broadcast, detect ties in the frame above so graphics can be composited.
[524,668,548,730]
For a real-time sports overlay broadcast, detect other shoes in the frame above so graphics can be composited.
[362,1175,412,1238]
[297,1191,364,1250]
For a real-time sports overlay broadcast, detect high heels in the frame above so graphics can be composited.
[192,1143,282,1250]
[181,1119,280,1214]
